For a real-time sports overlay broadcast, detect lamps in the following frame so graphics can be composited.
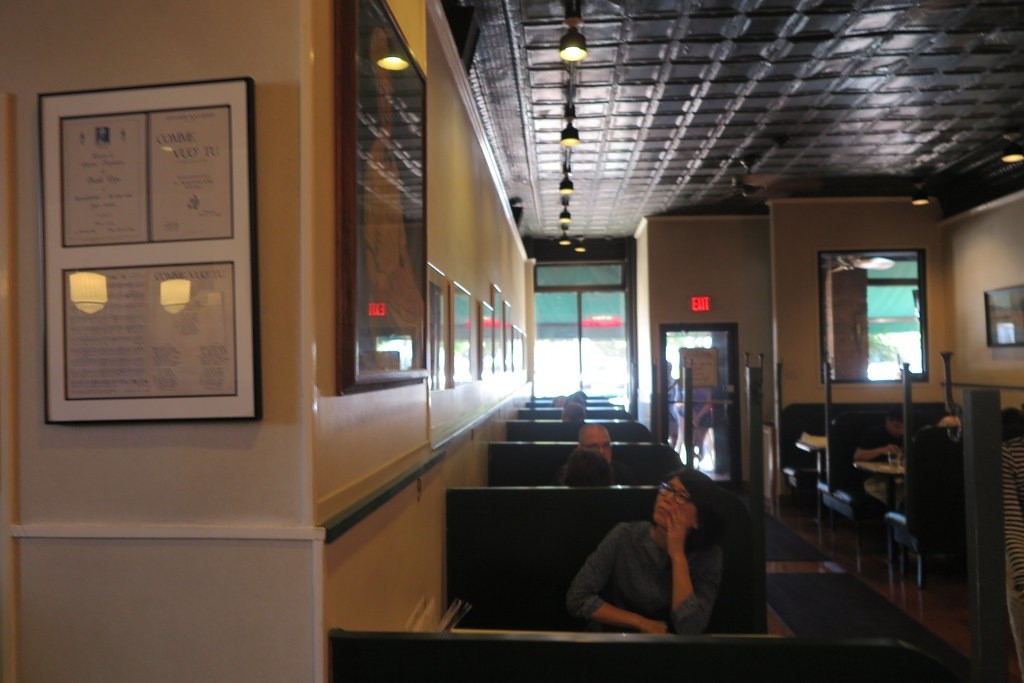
[559,163,574,195]
[1000,132,1024,161]
[559,225,572,246]
[573,237,587,252]
[559,197,571,223]
[911,182,929,205]
[561,105,579,147]
[560,0,588,62]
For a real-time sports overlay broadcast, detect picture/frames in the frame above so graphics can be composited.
[983,284,1024,348]
[333,0,430,394]
[37,76,261,425]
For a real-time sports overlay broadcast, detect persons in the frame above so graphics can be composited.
[577,423,637,485]
[854,409,906,507]
[567,467,723,636]
[665,363,678,449]
[691,387,712,459]
[561,449,609,489]
[552,392,587,440]
[1000,436,1024,680]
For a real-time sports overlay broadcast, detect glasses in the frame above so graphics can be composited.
[656,480,692,505]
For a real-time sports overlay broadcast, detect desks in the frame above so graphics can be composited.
[852,458,905,564]
[794,439,827,520]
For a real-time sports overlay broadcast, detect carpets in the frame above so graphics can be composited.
[765,572,971,683]
[752,500,829,562]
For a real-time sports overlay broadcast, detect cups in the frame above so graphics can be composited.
[888,451,901,467]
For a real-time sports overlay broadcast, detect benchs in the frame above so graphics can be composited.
[781,400,1024,590]
[328,396,958,683]
